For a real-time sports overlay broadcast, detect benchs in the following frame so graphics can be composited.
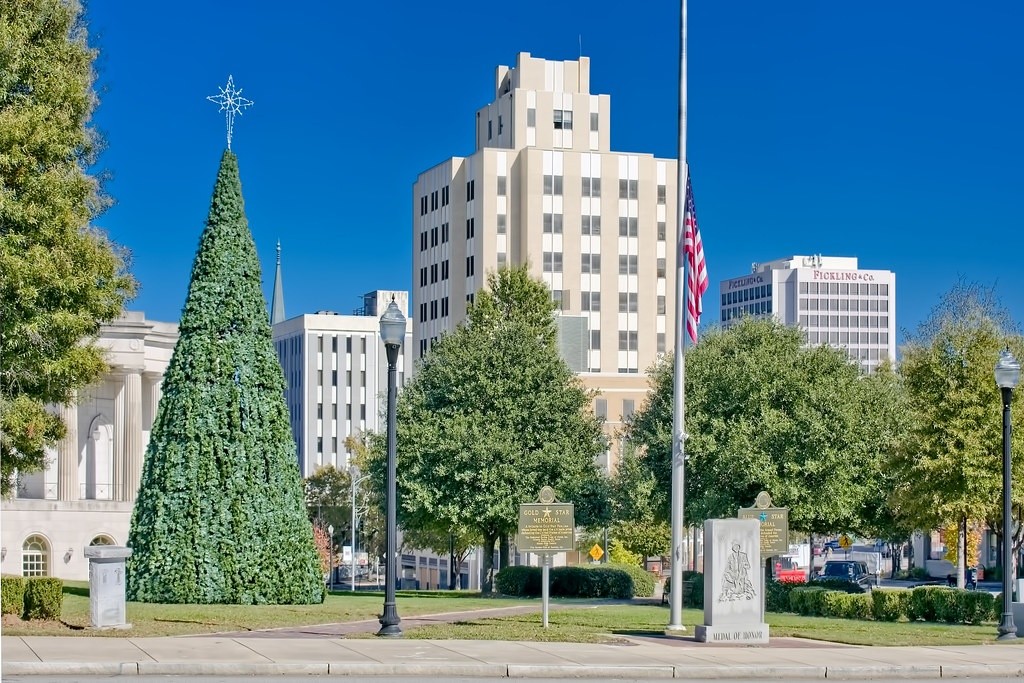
[662,580,695,607]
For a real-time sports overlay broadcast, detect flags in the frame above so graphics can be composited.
[684,162,709,345]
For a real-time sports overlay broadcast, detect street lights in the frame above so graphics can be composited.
[992,339,1023,641]
[327,524,335,591]
[377,293,408,639]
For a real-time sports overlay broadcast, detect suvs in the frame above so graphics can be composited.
[812,558,874,595]
